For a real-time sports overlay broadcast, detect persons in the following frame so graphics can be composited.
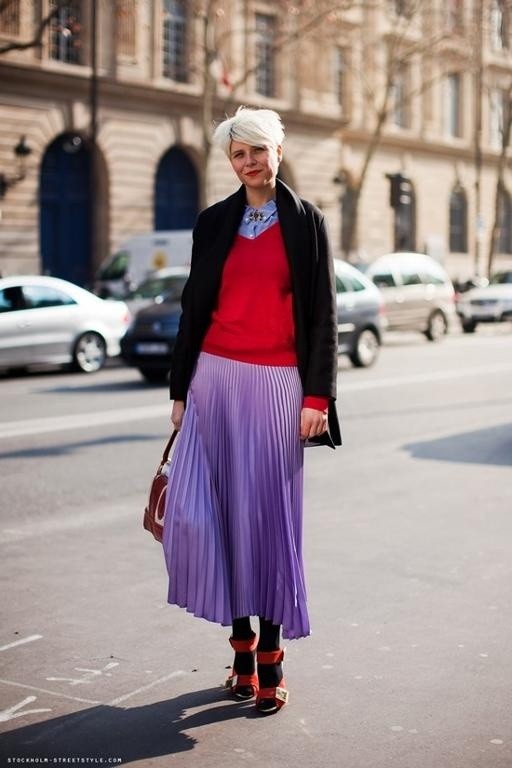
[157,102,346,719]
[50,9,83,65]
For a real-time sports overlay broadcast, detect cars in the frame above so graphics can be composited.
[120,256,384,385]
[451,268,512,333]
[0,274,130,376]
[120,265,191,317]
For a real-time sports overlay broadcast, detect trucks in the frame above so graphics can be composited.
[90,228,200,297]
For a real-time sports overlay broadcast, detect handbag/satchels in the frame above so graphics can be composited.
[141,427,182,545]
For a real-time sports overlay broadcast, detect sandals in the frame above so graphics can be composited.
[252,643,287,713]
[226,633,257,701]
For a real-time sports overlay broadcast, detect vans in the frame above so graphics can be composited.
[350,251,458,341]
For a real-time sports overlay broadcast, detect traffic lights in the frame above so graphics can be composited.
[397,170,413,208]
